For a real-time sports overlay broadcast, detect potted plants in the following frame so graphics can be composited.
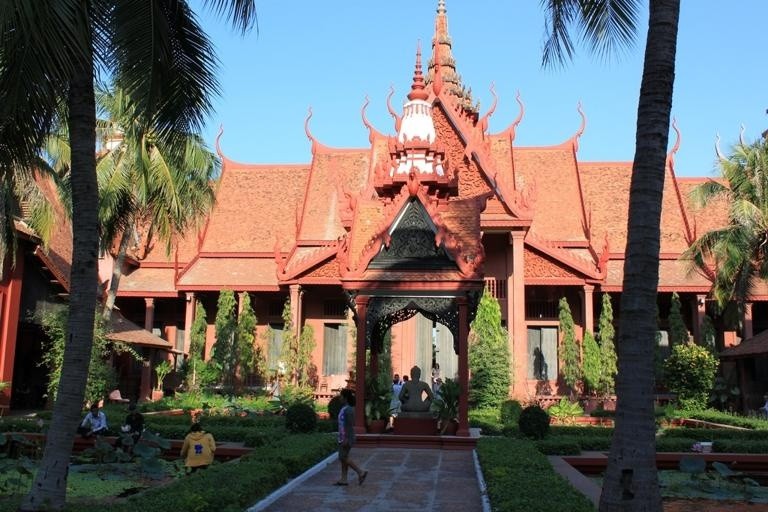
[364,363,394,434]
[151,360,172,403]
[431,376,464,435]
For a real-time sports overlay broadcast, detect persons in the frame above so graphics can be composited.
[80,403,107,438]
[399,366,434,412]
[388,374,408,417]
[759,394,768,414]
[331,388,368,486]
[270,376,281,402]
[116,404,146,454]
[432,363,440,391]
[180,422,216,473]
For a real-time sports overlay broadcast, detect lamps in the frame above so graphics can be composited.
[186,294,191,301]
[699,297,705,305]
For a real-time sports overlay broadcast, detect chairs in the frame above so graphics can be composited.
[320,378,328,392]
[0,404,10,418]
[108,389,129,406]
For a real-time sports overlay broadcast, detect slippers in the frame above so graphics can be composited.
[359,472,368,485]
[333,482,348,486]
